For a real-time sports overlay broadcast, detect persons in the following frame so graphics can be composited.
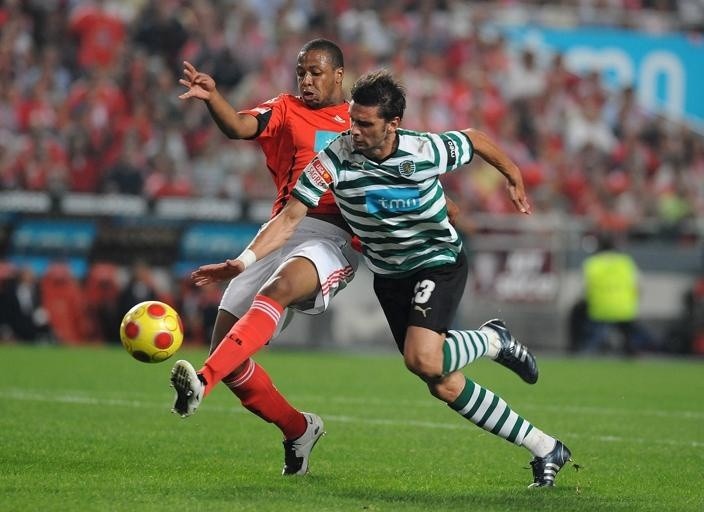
[189,70,575,490]
[0,258,225,346]
[582,236,641,338]
[168,37,461,478]
[0,1,703,239]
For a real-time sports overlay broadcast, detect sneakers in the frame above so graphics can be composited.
[170,360,206,416]
[282,413,326,475]
[477,319,538,384]
[527,441,571,489]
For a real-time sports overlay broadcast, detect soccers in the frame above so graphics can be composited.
[120,301,184,362]
